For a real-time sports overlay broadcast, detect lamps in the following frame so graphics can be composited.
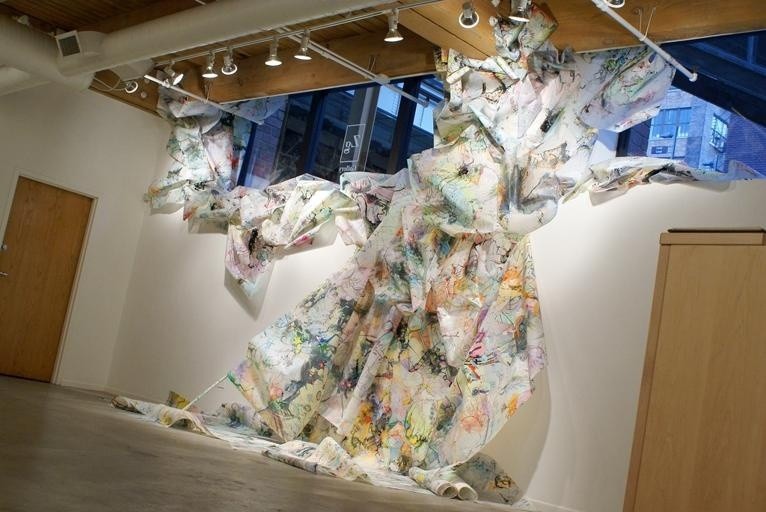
[120,0,630,95]
